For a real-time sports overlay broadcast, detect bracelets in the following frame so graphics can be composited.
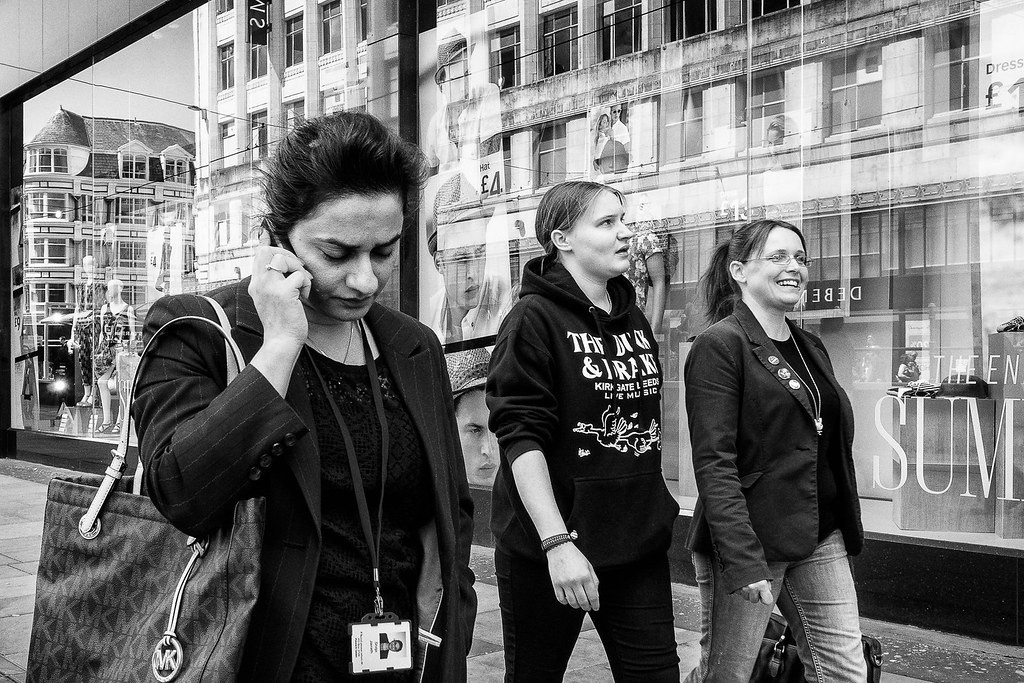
[540,530,577,551]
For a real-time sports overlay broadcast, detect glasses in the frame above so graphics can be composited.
[740,253,814,267]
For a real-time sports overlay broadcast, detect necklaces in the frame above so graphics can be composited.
[306,319,354,364]
[782,321,824,437]
[605,290,613,315]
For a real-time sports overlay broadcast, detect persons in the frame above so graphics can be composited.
[594,140,666,336]
[817,315,856,400]
[417,26,503,174]
[134,112,474,683]
[862,331,888,382]
[683,221,868,683]
[484,181,680,683]
[764,115,804,167]
[380,633,403,659]
[593,105,630,170]
[65,253,136,434]
[423,170,512,356]
[443,346,500,487]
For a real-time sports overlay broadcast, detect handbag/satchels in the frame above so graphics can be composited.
[24,297,264,683]
[749,612,883,683]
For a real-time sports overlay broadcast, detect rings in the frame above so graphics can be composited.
[266,265,282,273]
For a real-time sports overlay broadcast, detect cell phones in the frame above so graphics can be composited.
[256,214,296,278]
